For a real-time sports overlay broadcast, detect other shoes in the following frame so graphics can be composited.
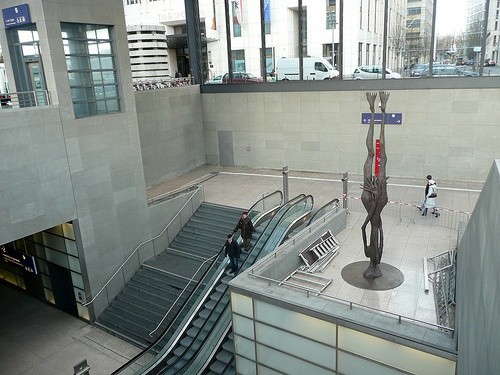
[229,271,237,276]
[244,248,250,252]
[417,207,441,218]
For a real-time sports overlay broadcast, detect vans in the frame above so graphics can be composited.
[277,57,339,81]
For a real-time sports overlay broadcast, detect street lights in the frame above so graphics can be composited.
[400,17,421,76]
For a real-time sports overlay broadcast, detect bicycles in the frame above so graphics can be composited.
[132,79,191,92]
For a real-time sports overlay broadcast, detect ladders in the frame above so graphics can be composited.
[278,228,341,297]
[424,250,456,333]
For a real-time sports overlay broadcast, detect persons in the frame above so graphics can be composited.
[175,71,182,78]
[270,69,275,77]
[234,211,254,251]
[224,234,241,275]
[417,175,440,217]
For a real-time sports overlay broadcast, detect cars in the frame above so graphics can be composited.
[428,58,495,67]
[204,74,222,84]
[409,64,426,77]
[353,65,403,80]
[223,72,263,84]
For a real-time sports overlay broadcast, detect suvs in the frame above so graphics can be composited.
[421,65,478,77]
